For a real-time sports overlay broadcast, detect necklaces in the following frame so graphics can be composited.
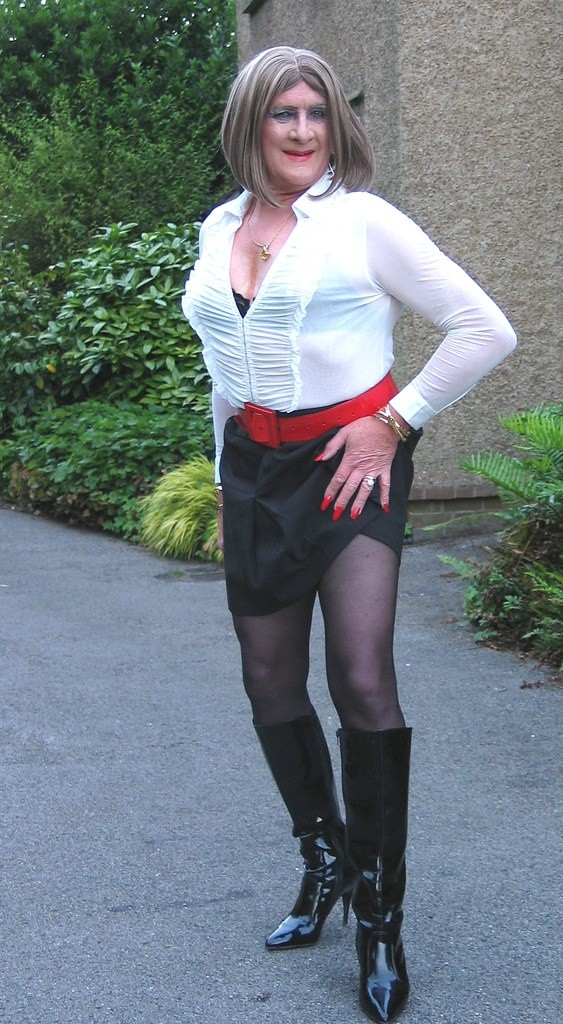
[247,200,294,261]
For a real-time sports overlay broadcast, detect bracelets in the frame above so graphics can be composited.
[372,403,411,443]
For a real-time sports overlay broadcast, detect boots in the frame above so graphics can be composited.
[335,726,410,1023]
[251,704,350,949]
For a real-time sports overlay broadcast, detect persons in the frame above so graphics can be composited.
[179,46,519,1024]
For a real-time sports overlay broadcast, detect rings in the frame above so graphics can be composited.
[362,475,375,487]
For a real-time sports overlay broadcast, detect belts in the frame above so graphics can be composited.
[233,370,399,450]
